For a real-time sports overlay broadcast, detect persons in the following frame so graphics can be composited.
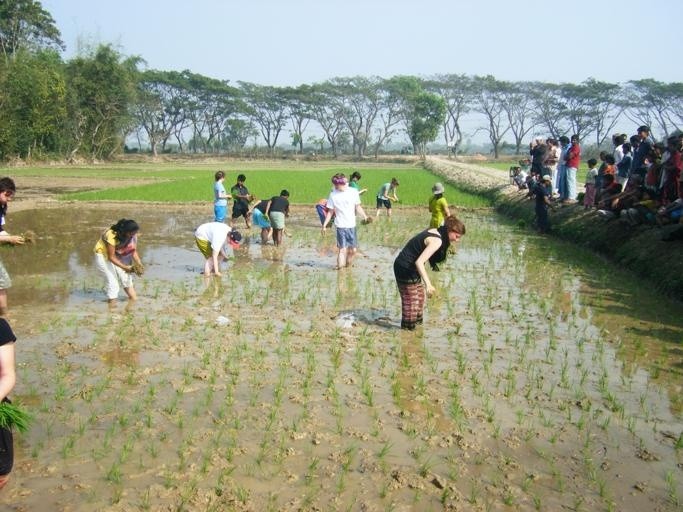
[349,172,368,218]
[214,172,232,222]
[429,183,450,230]
[93,218,141,304]
[0,177,24,313]
[320,173,368,270]
[514,125,683,228]
[246,200,271,241]
[264,190,290,246]
[195,222,241,278]
[393,215,466,330]
[231,175,254,228]
[316,199,335,228]
[1,317,16,488]
[376,177,399,216]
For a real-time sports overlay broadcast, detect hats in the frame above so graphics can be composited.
[542,174,551,182]
[431,183,444,195]
